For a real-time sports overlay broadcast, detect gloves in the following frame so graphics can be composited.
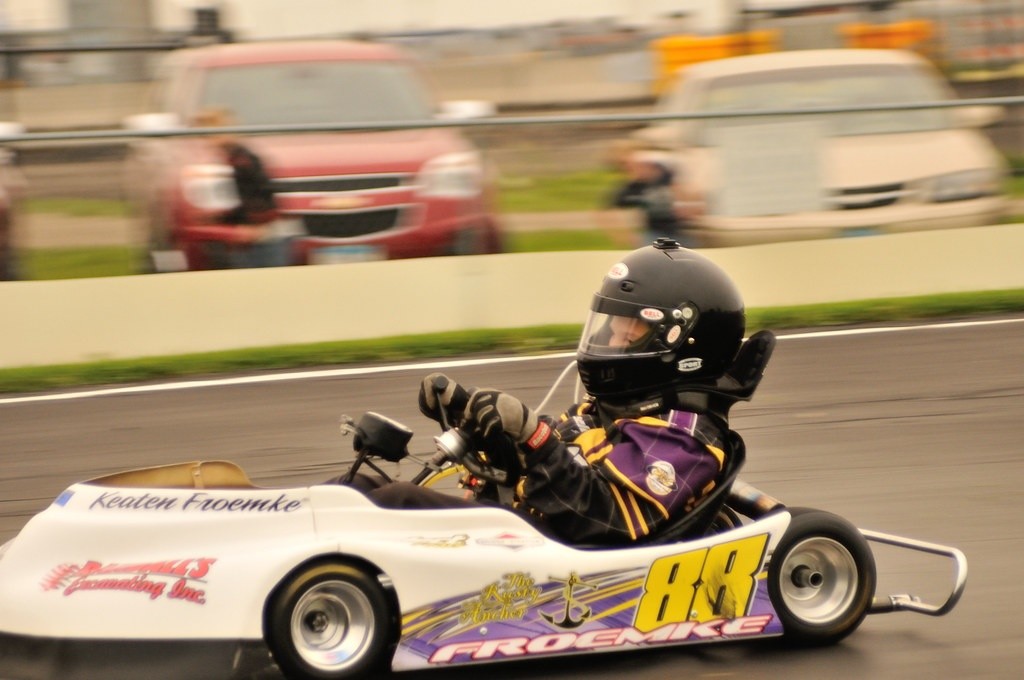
[418,372,470,424]
[460,388,539,449]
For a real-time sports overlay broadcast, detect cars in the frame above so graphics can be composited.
[616,47,1006,247]
[124,37,504,271]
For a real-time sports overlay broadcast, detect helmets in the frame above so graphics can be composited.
[576,237,744,403]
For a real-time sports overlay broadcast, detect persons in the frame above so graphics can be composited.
[322,236,744,546]
[223,140,278,226]
[608,150,684,236]
